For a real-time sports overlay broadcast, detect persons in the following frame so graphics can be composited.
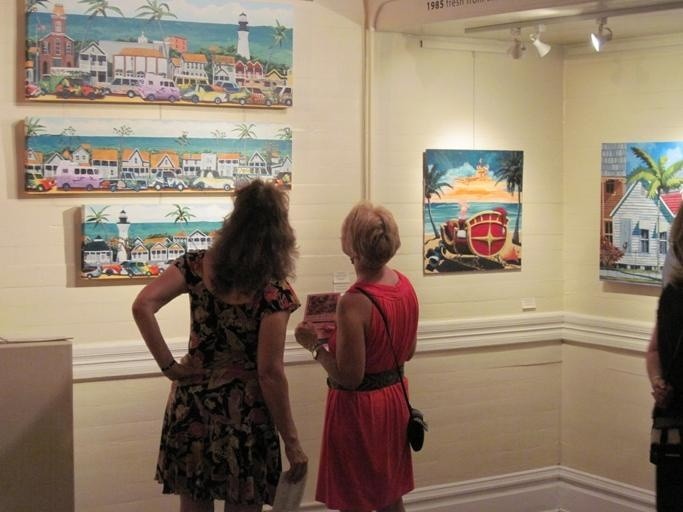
[129,174,309,511]
[642,200,683,510]
[292,198,421,511]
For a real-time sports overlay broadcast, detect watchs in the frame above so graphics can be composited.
[310,342,322,361]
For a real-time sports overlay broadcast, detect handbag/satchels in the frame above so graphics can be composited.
[648,413,681,470]
[406,410,429,452]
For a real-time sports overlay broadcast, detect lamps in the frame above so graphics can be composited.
[509,17,614,59]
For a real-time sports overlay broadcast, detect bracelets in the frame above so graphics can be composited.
[160,360,175,373]
[648,374,662,381]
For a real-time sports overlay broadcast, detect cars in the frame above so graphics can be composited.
[26,164,292,191]
[25,67,292,106]
[81,258,176,279]
